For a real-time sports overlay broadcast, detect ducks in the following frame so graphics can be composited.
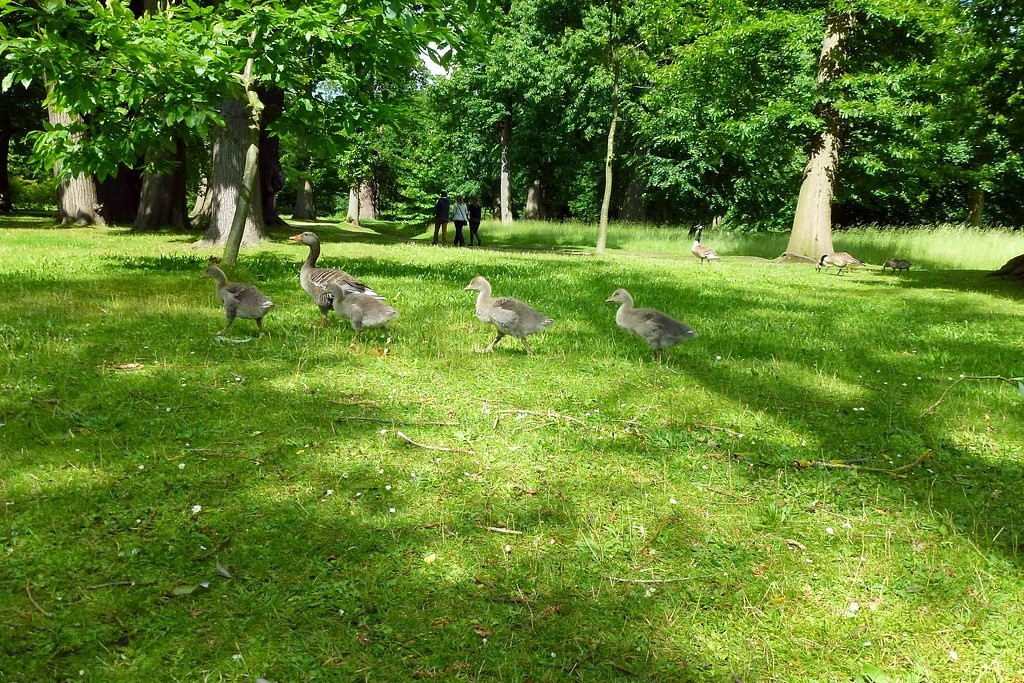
[604,287,698,364]
[691,223,719,264]
[320,283,398,345]
[200,265,275,339]
[288,232,388,329]
[814,253,912,276]
[463,275,554,355]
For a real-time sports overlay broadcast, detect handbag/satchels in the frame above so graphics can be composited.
[463,221,467,226]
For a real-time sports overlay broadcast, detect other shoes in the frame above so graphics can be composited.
[460,242,465,246]
[443,241,446,245]
[432,241,437,244]
[454,240,457,245]
[478,239,481,245]
[467,244,473,246]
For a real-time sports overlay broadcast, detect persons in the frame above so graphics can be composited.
[466,197,481,247]
[450,195,468,246]
[432,191,451,245]
